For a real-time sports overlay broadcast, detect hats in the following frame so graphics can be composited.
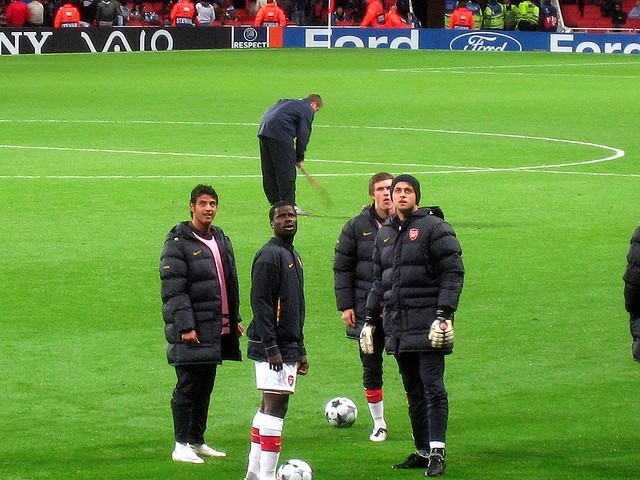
[389,174,421,204]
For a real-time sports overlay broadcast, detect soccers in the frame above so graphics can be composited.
[277,459,313,480]
[325,397,357,428]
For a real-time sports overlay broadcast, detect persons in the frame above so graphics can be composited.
[27,1,43,24]
[257,94,322,215]
[80,0,96,27]
[610,2,627,26]
[159,186,242,464]
[213,1,241,22]
[291,0,409,25]
[538,0,558,31]
[502,0,519,30]
[444,4,455,29]
[465,0,483,29]
[361,174,465,478]
[517,0,539,31]
[195,1,216,27]
[255,0,278,12]
[95,0,116,25]
[252,0,285,26]
[5,1,31,26]
[42,0,55,25]
[53,1,80,28]
[447,5,475,30]
[116,0,170,27]
[171,0,194,26]
[622,223,640,364]
[483,0,504,30]
[244,204,308,479]
[333,172,397,441]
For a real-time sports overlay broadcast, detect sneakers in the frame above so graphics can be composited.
[188,444,226,457]
[171,444,205,464]
[294,205,309,215]
[391,450,429,470]
[369,428,387,442]
[426,448,446,477]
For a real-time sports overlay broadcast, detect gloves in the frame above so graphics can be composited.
[427,317,455,354]
[360,322,376,354]
